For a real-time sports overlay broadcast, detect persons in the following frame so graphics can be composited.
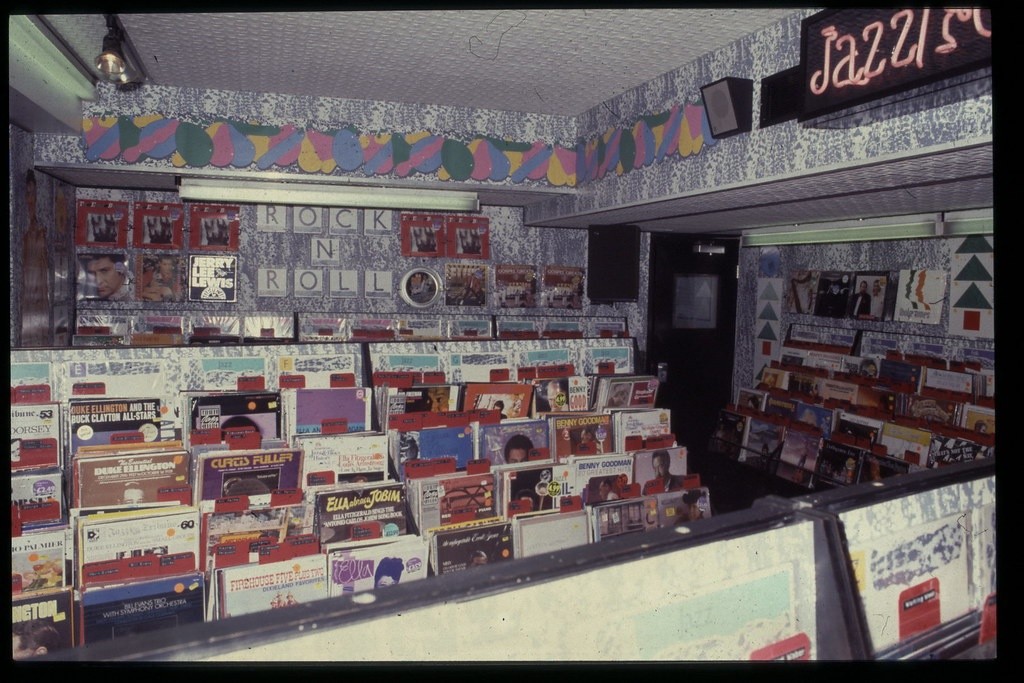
[597,478,620,502]
[795,441,810,474]
[11,438,28,464]
[829,273,851,313]
[747,395,761,410]
[851,279,884,318]
[419,385,451,413]
[495,278,535,307]
[142,254,182,302]
[491,399,510,420]
[680,489,708,521]
[608,384,627,407]
[974,419,988,434]
[545,380,568,412]
[113,481,149,504]
[399,432,420,465]
[516,489,537,512]
[13,618,66,659]
[372,558,405,592]
[350,475,369,484]
[643,450,685,491]
[77,254,136,306]
[545,280,584,307]
[759,443,772,463]
[578,426,604,454]
[409,271,432,298]
[270,589,299,608]
[467,550,490,569]
[503,432,537,464]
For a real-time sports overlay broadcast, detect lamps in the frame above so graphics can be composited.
[94,17,146,93]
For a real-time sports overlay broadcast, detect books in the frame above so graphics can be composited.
[11,369,709,658]
[711,305,994,506]
